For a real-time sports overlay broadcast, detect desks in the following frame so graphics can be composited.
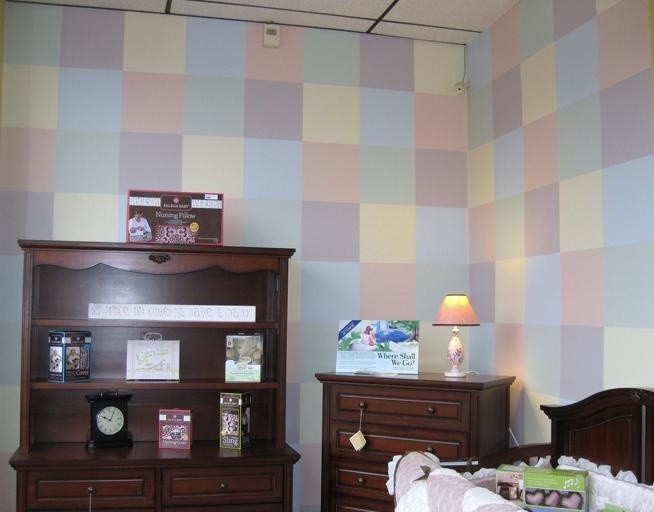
[385,449,653,511]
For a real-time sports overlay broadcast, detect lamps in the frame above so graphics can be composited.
[430,294,480,378]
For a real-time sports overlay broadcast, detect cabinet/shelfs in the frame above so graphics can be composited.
[314,371,518,511]
[27,470,156,512]
[162,464,286,512]
[16,238,296,454]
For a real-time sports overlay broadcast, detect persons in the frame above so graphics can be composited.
[128,208,152,242]
[362,326,377,345]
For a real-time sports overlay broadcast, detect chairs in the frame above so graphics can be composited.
[538,387,652,486]
